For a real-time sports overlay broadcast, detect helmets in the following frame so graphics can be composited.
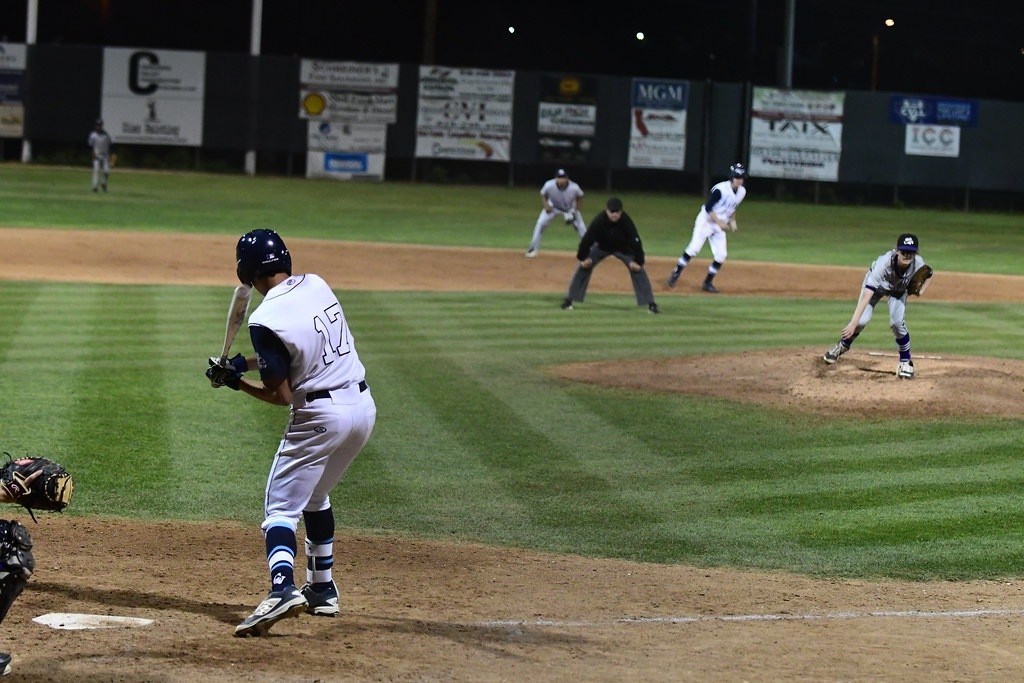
[728,162,749,179]
[235,228,292,289]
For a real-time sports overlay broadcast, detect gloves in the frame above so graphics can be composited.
[205,353,248,391]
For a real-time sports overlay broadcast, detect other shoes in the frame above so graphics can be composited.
[647,303,658,315]
[667,276,676,289]
[524,246,537,258]
[702,281,720,293]
[560,298,574,310]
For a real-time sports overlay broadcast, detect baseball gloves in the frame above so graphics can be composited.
[908,264,933,297]
[0,455,74,512]
[563,207,577,223]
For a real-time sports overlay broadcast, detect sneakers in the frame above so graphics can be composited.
[823,338,850,364]
[298,577,341,616]
[896,357,914,378]
[235,584,310,639]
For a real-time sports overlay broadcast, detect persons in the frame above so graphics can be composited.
[206,228,376,637]
[89,119,111,193]
[525,169,587,258]
[824,234,933,377]
[0,452,73,677]
[667,162,746,293]
[561,198,661,314]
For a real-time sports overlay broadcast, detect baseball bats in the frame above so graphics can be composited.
[209,287,251,388]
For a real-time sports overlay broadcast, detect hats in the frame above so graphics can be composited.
[607,197,622,212]
[555,166,568,177]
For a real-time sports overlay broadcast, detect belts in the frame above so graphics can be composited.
[305,380,368,402]
